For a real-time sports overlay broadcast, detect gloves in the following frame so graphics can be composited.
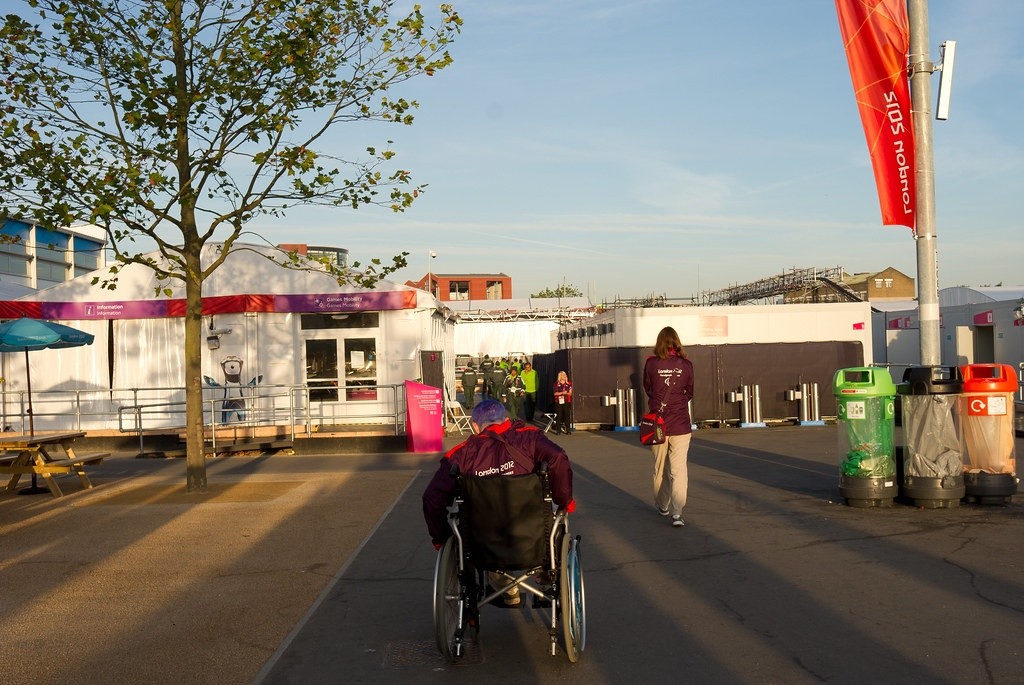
[564,498,576,513]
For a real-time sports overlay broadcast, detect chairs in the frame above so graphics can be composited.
[543,412,564,434]
[446,401,475,435]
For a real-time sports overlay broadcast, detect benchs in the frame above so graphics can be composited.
[0,455,32,467]
[38,452,111,474]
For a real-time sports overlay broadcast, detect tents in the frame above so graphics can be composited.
[0,241,464,430]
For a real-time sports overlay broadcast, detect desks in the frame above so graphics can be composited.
[0,432,93,498]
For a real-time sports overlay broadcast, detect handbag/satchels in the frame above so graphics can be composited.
[640,413,666,445]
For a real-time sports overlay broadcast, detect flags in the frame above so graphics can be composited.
[834,0,917,233]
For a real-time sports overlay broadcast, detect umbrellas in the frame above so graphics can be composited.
[0,314,95,490]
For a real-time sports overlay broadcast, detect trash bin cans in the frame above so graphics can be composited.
[955,362,1019,506]
[896,365,964,509]
[831,366,898,509]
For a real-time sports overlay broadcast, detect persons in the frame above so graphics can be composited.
[479,354,525,400]
[502,366,526,420]
[462,361,479,408]
[554,371,573,436]
[422,400,576,606]
[520,362,539,422]
[642,326,694,526]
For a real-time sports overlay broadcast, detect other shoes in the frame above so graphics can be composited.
[536,583,554,601]
[502,587,520,605]
[671,514,685,526]
[657,507,670,516]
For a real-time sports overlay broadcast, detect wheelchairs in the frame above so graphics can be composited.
[430,505,589,667]
